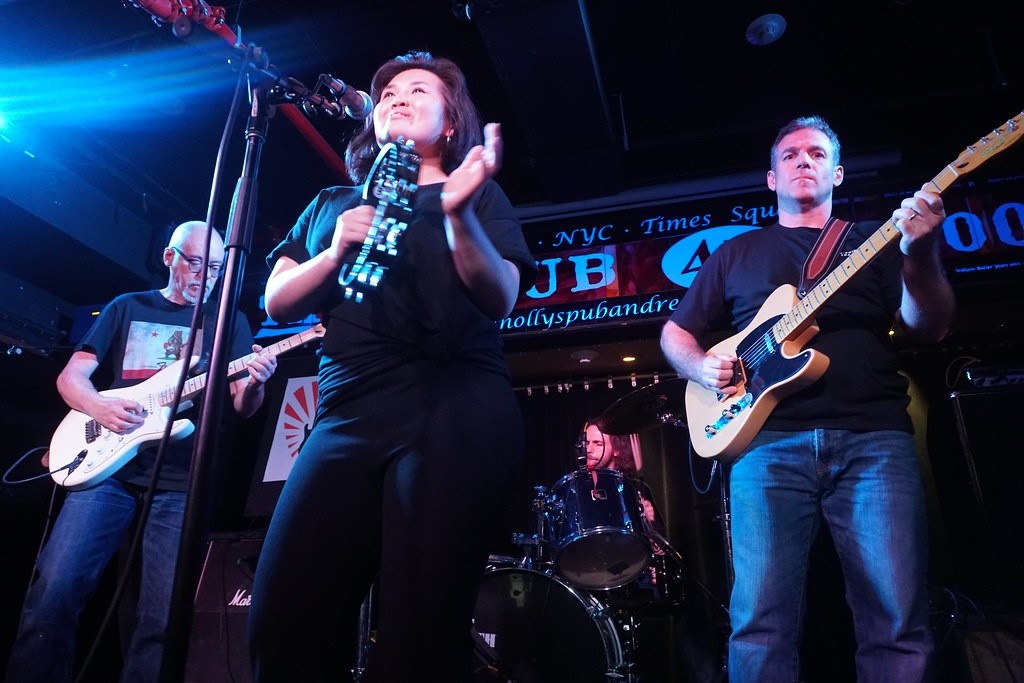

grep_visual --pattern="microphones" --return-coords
[318,73,373,120]
[580,422,588,465]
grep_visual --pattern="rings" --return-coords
[907,212,919,222]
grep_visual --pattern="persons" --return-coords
[660,116,955,683]
[8,219,278,682]
[244,52,536,683]
[581,418,657,527]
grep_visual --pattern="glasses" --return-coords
[170,247,224,278]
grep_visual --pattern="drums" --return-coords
[541,466,654,593]
[469,565,625,683]
[484,554,521,574]
[510,531,545,562]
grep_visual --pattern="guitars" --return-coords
[681,106,1024,469]
[46,323,326,494]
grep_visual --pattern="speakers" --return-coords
[927,384,1024,613]
[183,529,266,683]
